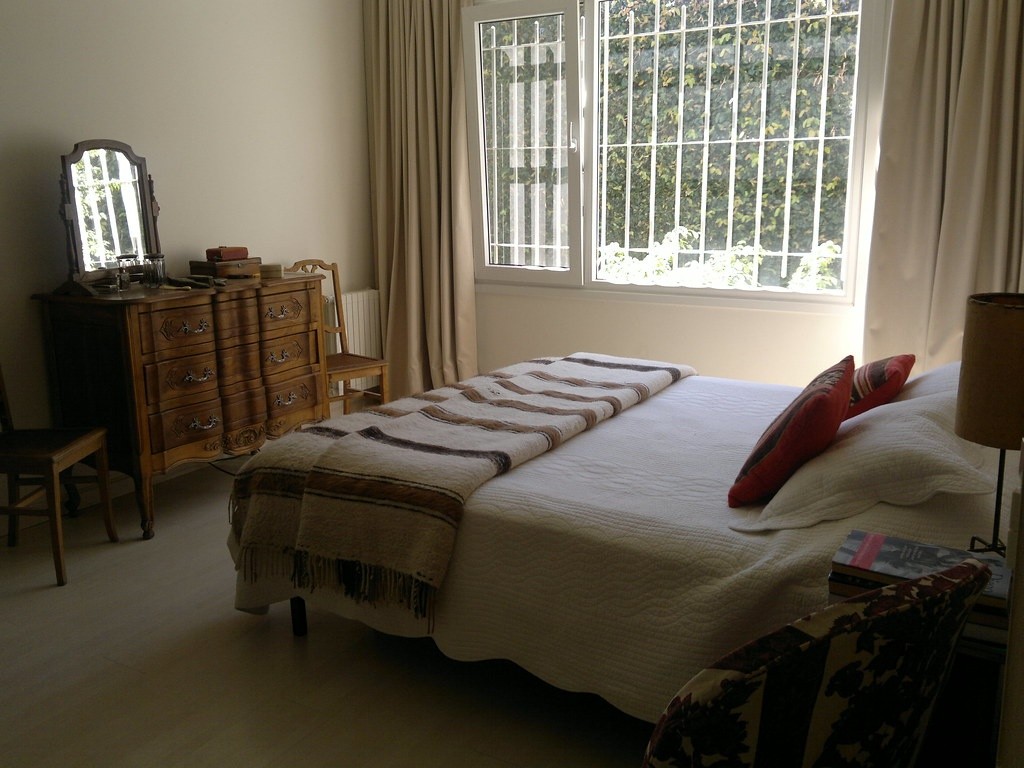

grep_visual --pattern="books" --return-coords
[826,527,1010,606]
[826,571,1009,630]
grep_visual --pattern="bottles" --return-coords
[116,268,131,293]
[143,253,165,289]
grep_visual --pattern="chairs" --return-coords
[643,557,995,767]
[1,377,121,584]
[283,259,389,416]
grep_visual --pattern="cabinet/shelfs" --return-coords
[30,269,331,539]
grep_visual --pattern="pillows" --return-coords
[729,355,855,509]
[894,359,963,403]
[842,354,917,421]
[727,387,996,532]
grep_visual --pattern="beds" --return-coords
[226,352,1024,727]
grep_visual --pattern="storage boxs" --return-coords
[190,246,262,279]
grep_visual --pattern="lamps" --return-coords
[956,291,1024,560]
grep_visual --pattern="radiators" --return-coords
[324,289,381,397]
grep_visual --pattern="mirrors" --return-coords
[61,139,164,284]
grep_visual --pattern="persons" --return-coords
[841,530,1005,585]
